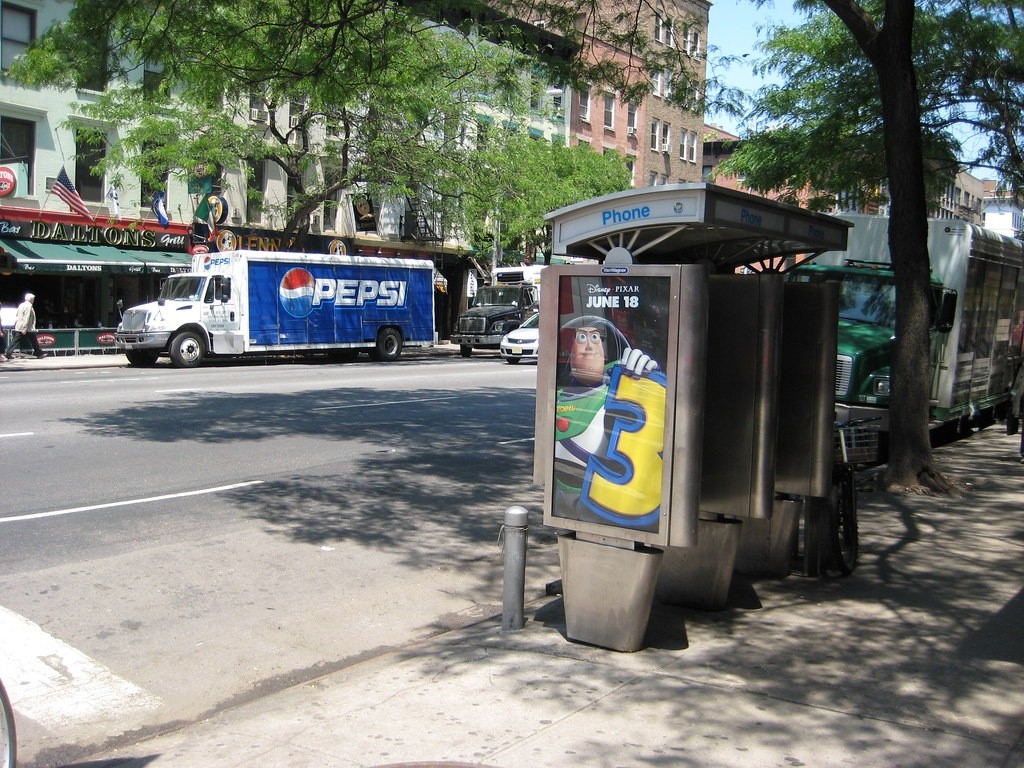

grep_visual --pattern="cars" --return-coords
[500,312,539,364]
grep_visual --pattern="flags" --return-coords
[51,166,95,224]
[193,191,219,243]
[152,188,169,229]
[106,185,123,221]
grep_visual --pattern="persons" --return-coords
[0,303,9,362]
[3,293,48,359]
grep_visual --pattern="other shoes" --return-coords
[37,354,47,359]
[5,354,14,360]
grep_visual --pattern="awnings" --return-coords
[0,236,197,277]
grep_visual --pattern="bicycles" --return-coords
[831,415,883,575]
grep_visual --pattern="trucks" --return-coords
[786,212,1023,450]
[114,249,438,369]
[450,264,547,358]
